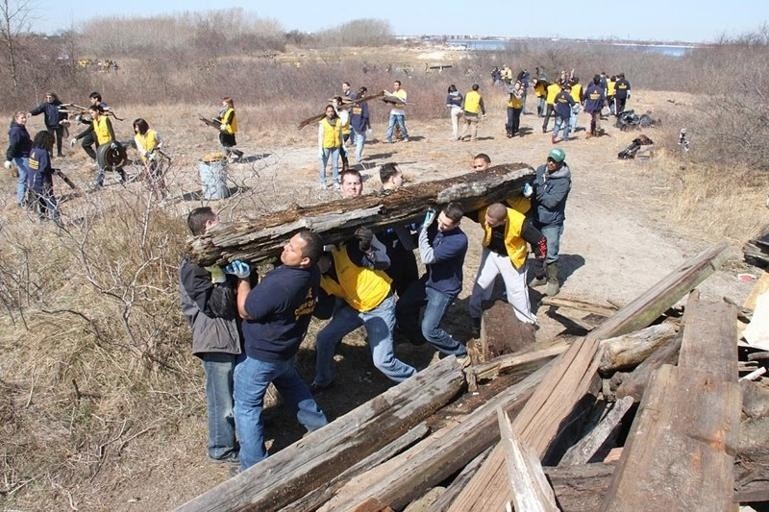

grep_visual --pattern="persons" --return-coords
[461,84,486,141]
[446,84,464,141]
[213,97,244,164]
[4,90,165,229]
[381,80,409,144]
[332,97,350,172]
[490,64,631,143]
[342,81,358,144]
[318,105,342,189]
[350,88,372,162]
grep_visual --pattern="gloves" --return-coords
[423,209,436,228]
[71,138,77,148]
[204,260,250,283]
[5,161,12,169]
[524,183,533,197]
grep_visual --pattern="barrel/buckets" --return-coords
[198,154,228,202]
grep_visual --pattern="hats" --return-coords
[548,148,565,163]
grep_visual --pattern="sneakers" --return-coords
[528,277,559,297]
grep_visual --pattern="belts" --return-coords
[366,280,396,312]
